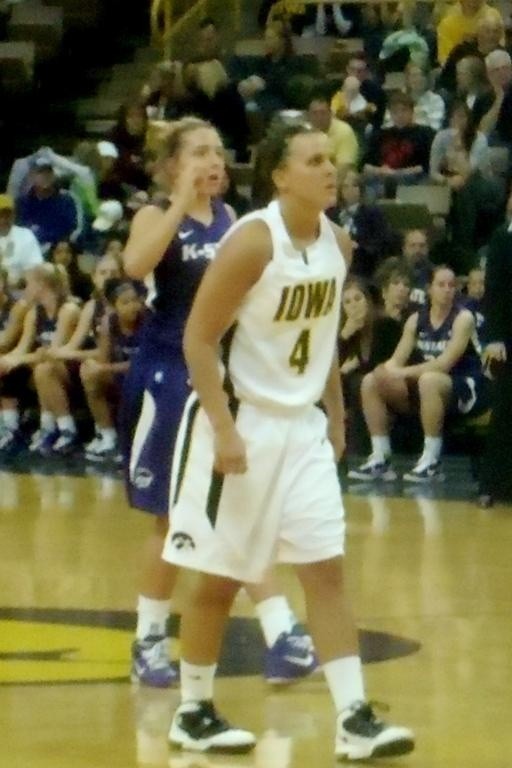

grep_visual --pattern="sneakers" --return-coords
[346,454,395,480]
[0,426,117,462]
[403,460,445,483]
[131,642,178,686]
[335,707,415,761]
[264,634,319,685]
[167,699,256,754]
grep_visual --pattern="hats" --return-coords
[92,200,126,233]
[30,147,60,169]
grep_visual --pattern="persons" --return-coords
[1,1,512,504]
[117,112,325,688]
[160,124,415,766]
